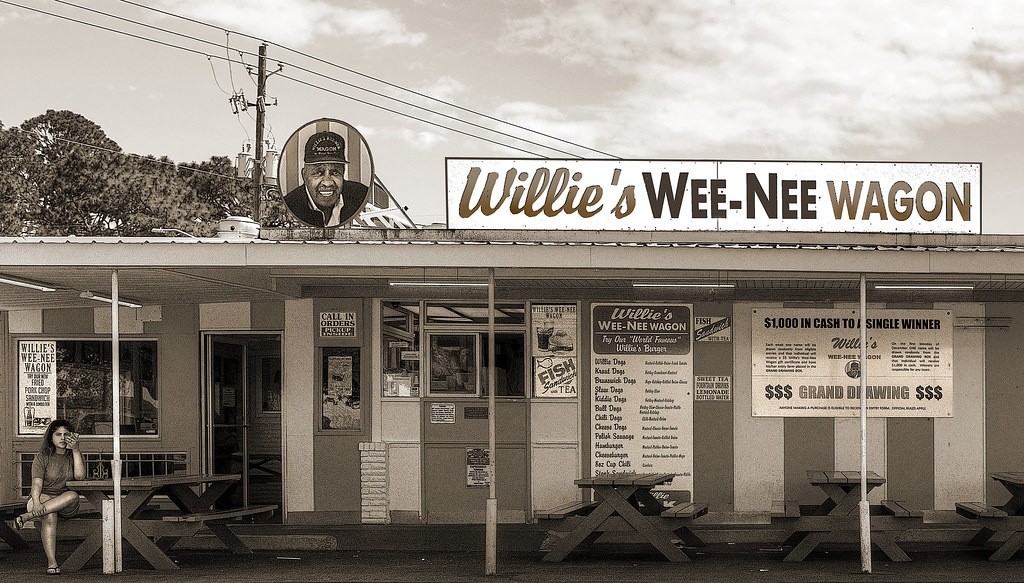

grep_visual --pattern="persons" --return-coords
[14,419,87,574]
[284,131,368,227]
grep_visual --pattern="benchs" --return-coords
[34,505,160,539]
[955,502,1009,525]
[770,500,801,531]
[533,501,600,531]
[162,503,284,538]
[661,502,709,530]
[881,501,924,530]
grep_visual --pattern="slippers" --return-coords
[46,567,62,575]
[14,515,25,531]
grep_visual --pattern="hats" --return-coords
[304,131,351,166]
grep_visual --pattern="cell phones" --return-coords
[66,432,79,449]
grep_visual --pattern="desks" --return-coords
[59,473,242,573]
[542,474,707,563]
[779,471,912,562]
[966,472,1024,562]
